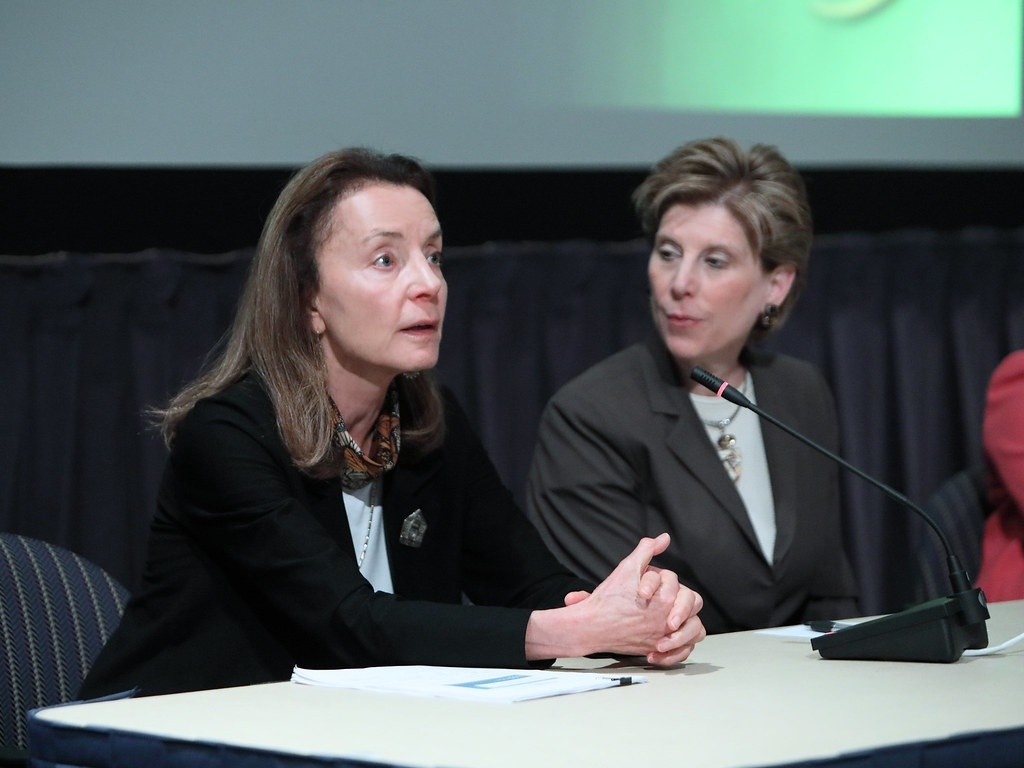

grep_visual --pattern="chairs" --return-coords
[0,531,134,768]
[918,463,1000,601]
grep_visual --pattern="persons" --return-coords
[523,139,859,634]
[972,349,1024,603]
[75,147,706,703]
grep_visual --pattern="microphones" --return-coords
[689,362,993,666]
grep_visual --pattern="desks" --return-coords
[28,599,1024,768]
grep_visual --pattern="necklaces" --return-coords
[703,372,747,450]
[358,480,376,568]
